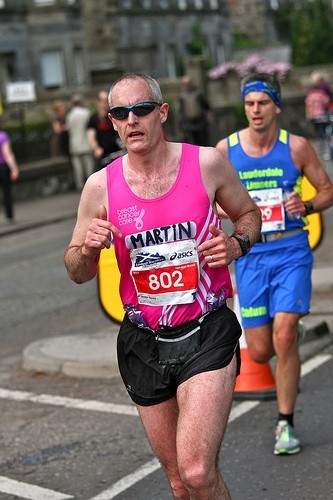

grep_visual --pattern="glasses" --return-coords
[108,100,162,120]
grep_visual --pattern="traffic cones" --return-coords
[228,288,279,400]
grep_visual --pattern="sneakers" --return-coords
[298,320,305,345]
[273,420,301,455]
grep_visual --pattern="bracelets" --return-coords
[303,201,314,217]
[230,232,251,257]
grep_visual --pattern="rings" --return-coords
[211,255,212,262]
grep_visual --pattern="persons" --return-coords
[176,75,215,146]
[0,113,19,226]
[64,92,104,190]
[303,70,332,159]
[212,73,333,455]
[84,89,124,172]
[64,72,262,500]
[49,98,67,157]
